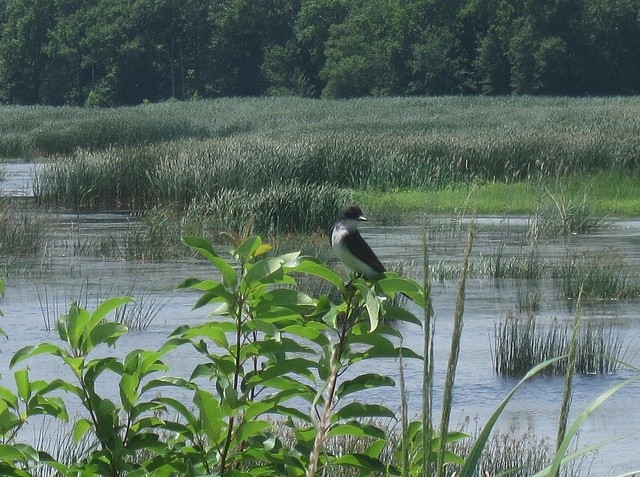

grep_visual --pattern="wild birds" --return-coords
[330,206,417,302]
